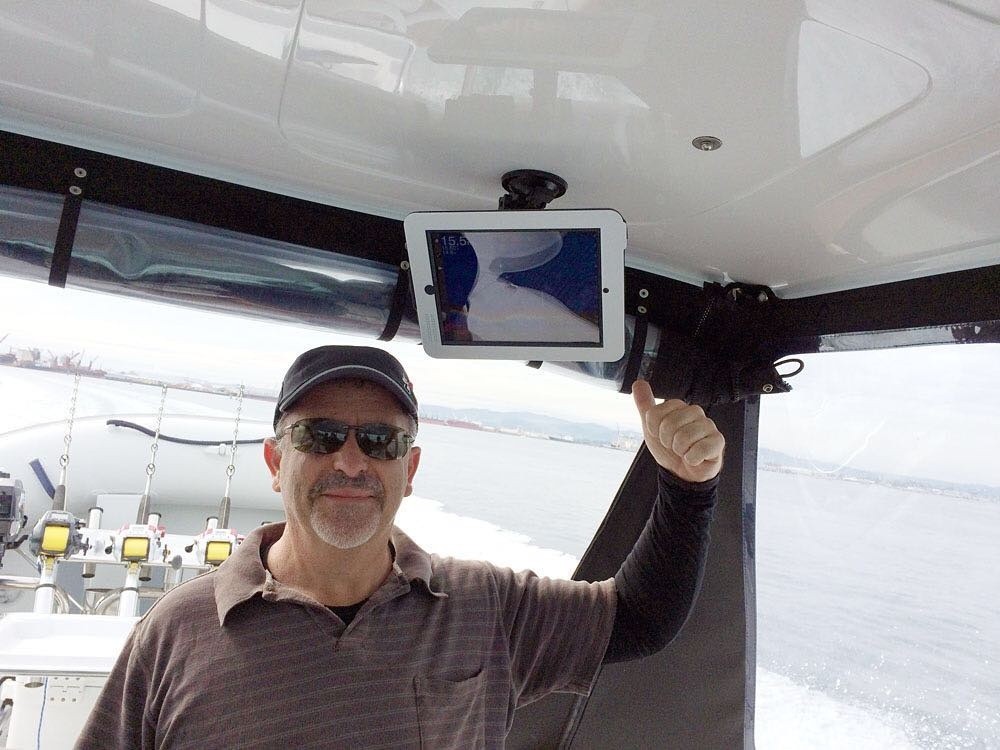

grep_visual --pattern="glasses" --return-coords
[282,416,415,461]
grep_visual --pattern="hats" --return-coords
[272,344,418,435]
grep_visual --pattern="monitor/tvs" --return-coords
[403,207,624,361]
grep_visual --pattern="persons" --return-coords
[74,345,725,750]
